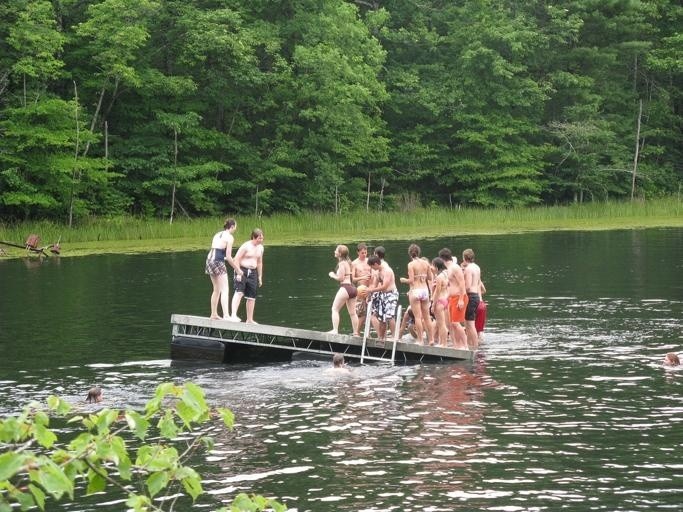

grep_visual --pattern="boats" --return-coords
[170,313,473,363]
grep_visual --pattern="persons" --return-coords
[204,216,245,324]
[327,353,349,371]
[324,240,486,353]
[662,352,681,365]
[85,385,103,404]
[228,228,264,327]
